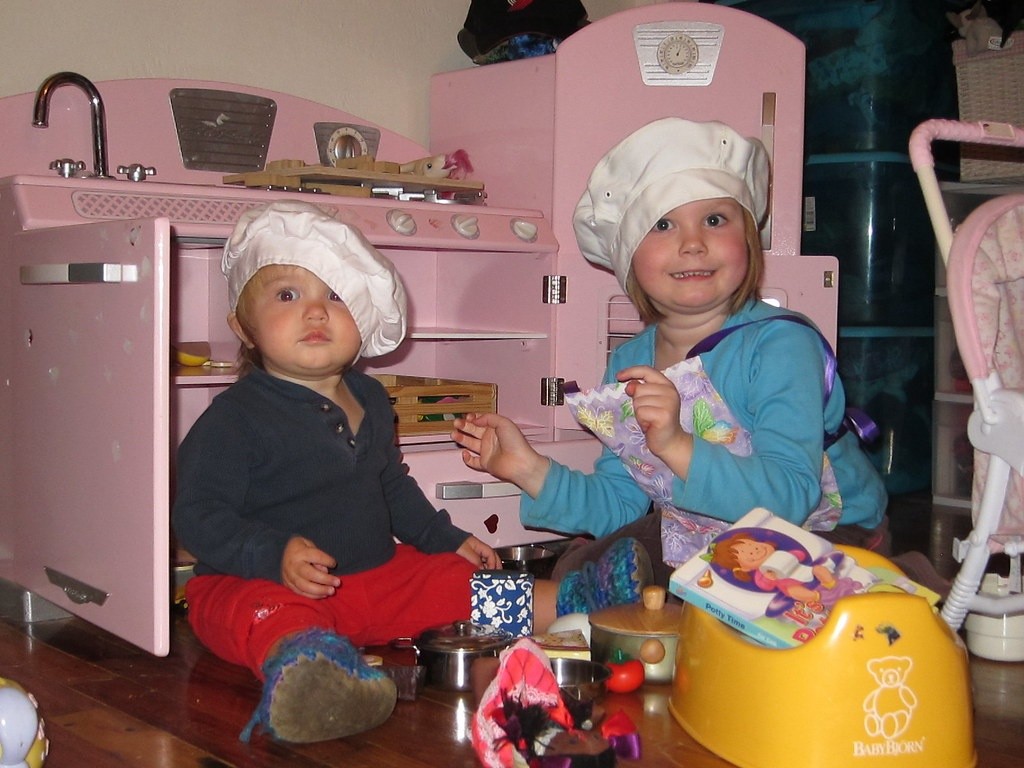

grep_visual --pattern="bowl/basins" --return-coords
[547,656,613,714]
[490,544,558,583]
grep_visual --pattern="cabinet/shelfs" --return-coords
[721,0,968,491]
[930,180,1024,581]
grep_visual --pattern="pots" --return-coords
[389,619,515,692]
[588,586,682,686]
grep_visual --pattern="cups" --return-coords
[469,568,535,640]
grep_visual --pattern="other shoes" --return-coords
[556,536,655,616]
[238,626,399,746]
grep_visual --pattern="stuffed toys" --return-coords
[469,636,641,768]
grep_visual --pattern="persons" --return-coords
[449,118,955,602]
[170,198,656,743]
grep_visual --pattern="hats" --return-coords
[220,199,407,368]
[572,116,770,298]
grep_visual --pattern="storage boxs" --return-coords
[368,373,499,437]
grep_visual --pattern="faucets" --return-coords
[30,71,157,180]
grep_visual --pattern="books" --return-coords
[668,506,943,651]
